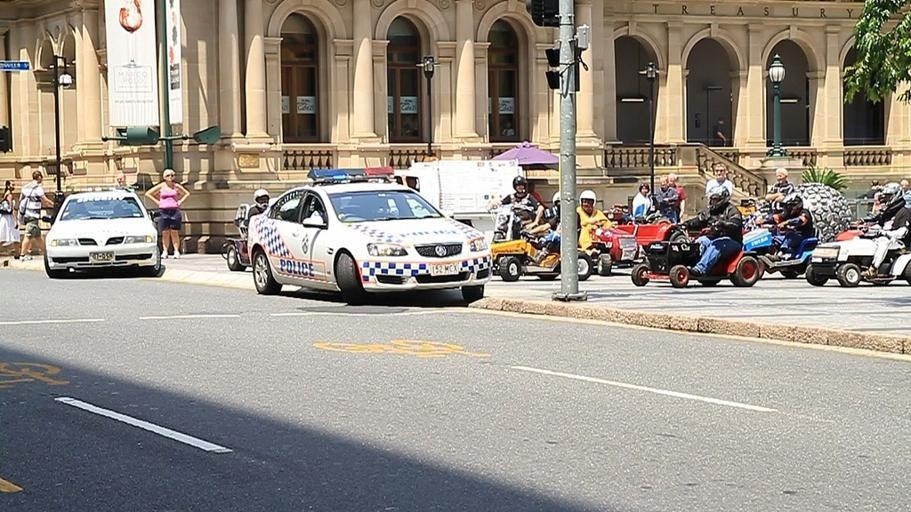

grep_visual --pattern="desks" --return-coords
[0,124,11,154]
[51,56,73,221]
[767,49,787,156]
[416,53,440,158]
[639,59,663,194]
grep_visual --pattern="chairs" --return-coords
[173,251,180,258]
[161,252,168,258]
[688,266,700,276]
[861,265,878,278]
[766,254,779,261]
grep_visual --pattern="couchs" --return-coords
[545,45,581,94]
[525,0,558,27]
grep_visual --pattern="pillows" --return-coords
[253,189,269,209]
[878,182,903,211]
[707,186,730,211]
[780,196,803,219]
[513,176,526,187]
[553,192,560,205]
[580,190,596,206]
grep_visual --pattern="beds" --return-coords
[491,141,581,179]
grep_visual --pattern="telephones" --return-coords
[0,60,31,71]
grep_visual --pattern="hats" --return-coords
[42,186,166,281]
[245,164,493,305]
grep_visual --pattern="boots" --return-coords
[220,199,259,275]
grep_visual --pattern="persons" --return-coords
[317,197,346,222]
[850,179,910,279]
[609,208,627,225]
[245,186,271,220]
[671,185,743,278]
[527,191,561,264]
[631,183,653,224]
[705,164,732,198]
[650,177,676,223]
[713,116,728,147]
[12,171,54,261]
[668,173,687,223]
[144,168,191,260]
[0,179,21,260]
[741,169,818,262]
[503,122,516,137]
[491,176,543,242]
[577,190,613,260]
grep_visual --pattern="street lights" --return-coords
[17,198,28,225]
[0,200,15,214]
